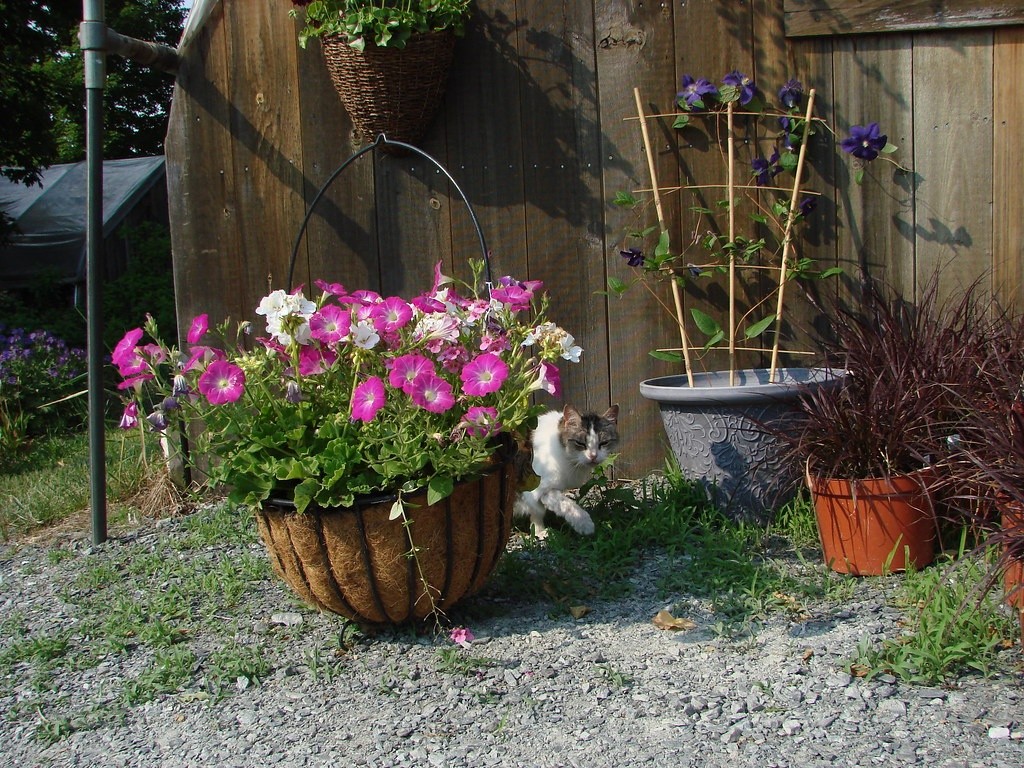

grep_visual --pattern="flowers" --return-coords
[110,254,585,648]
[591,68,919,367]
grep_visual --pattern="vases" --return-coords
[638,368,852,529]
[248,438,518,635]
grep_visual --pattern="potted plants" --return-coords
[703,343,964,578]
[896,389,1024,609]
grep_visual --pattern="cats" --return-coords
[513,403,621,538]
